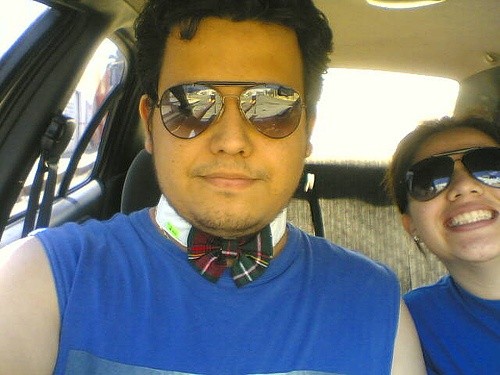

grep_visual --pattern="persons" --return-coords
[0,0,427,375]
[388,116,499,375]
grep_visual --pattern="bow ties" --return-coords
[183,226,275,287]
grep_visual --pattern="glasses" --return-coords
[407,146,500,202]
[155,80,309,139]
